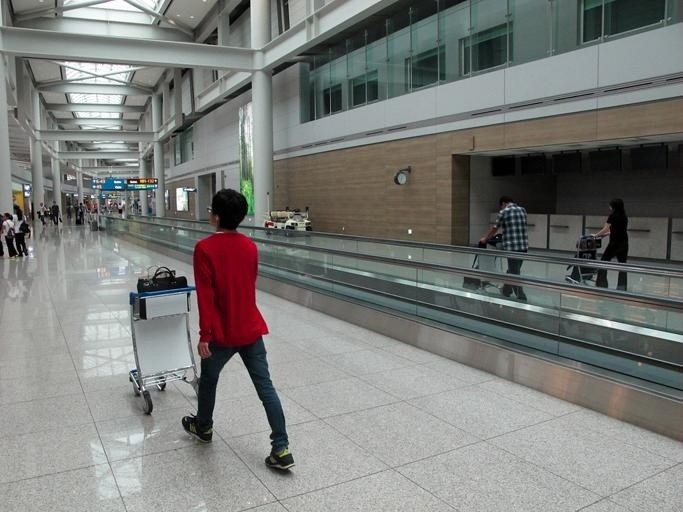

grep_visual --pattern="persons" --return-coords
[16,257,31,304]
[51,201,60,225]
[1,213,17,260]
[478,194,528,302]
[51,226,61,249]
[65,199,140,225]
[181,187,295,471]
[38,226,48,250]
[593,198,629,291]
[0,214,4,256]
[78,226,87,248]
[0,256,5,299]
[5,258,18,304]
[11,204,28,257]
[36,202,46,226]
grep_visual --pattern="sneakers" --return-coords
[9,249,29,258]
[264,446,297,471]
[181,413,213,444]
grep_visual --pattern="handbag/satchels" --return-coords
[575,236,602,249]
[19,220,30,234]
[478,233,505,249]
[136,264,188,321]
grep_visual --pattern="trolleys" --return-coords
[463,235,504,295]
[128,286,200,414]
[565,234,604,287]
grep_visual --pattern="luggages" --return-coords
[570,254,599,282]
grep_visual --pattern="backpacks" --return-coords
[5,220,16,240]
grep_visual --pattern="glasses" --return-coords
[204,202,216,214]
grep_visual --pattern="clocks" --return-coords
[393,170,407,185]
